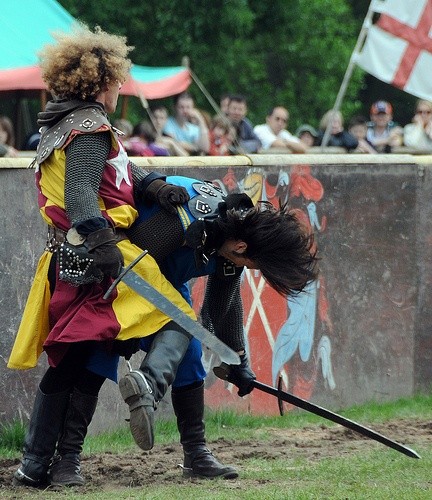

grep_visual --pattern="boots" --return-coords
[14,383,70,486]
[119,330,190,451]
[47,392,98,485]
[171,381,240,479]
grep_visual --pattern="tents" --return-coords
[0,0,246,156]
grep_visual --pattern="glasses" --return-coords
[415,109,432,115]
[272,114,288,126]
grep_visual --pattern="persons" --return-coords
[7,27,199,488]
[0,94,431,156]
[50,175,324,485]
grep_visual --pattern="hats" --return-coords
[370,101,392,115]
[295,123,319,139]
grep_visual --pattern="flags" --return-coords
[356,0,432,102]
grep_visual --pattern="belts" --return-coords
[46,226,69,245]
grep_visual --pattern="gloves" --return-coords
[116,339,140,361]
[146,177,190,214]
[222,351,256,397]
[76,217,124,281]
